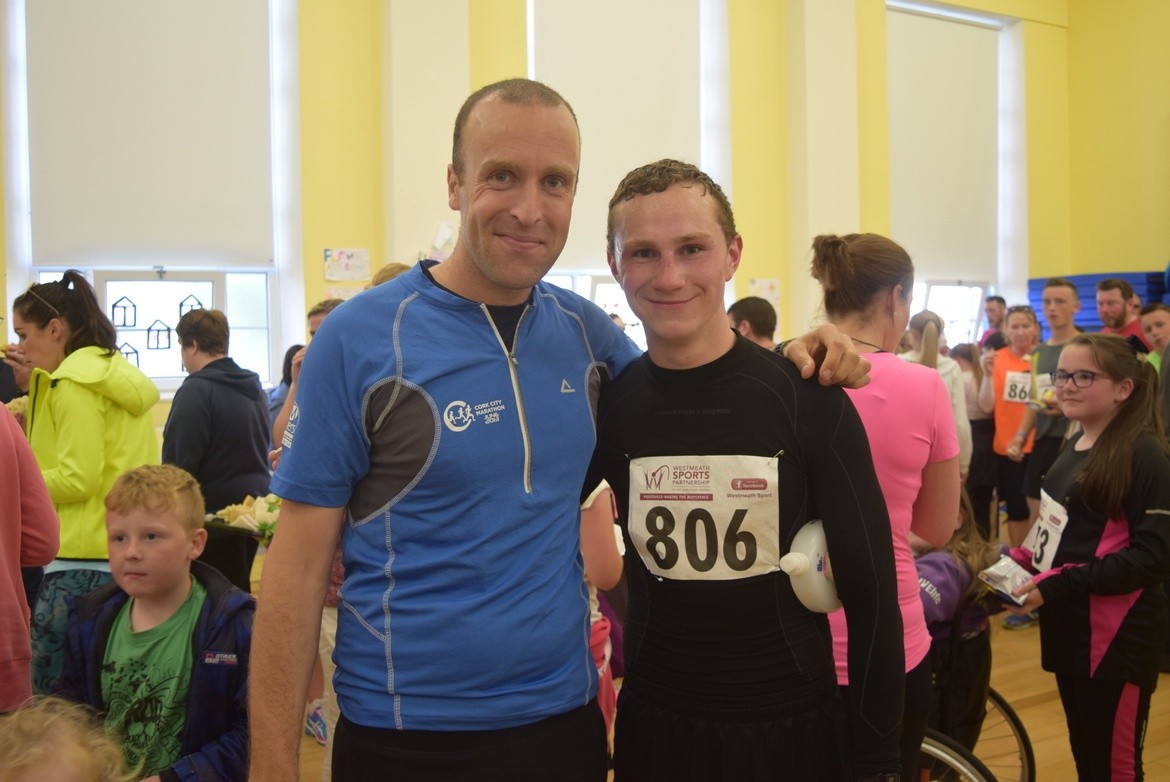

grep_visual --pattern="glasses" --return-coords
[1008,306,1033,313]
[1049,368,1121,389]
[24,282,62,322]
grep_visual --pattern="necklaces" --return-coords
[852,338,882,352]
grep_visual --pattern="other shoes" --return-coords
[997,500,1008,523]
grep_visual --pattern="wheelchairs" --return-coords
[914,646,1036,782]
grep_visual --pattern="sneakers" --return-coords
[1002,612,1039,631]
[304,707,327,746]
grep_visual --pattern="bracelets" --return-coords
[774,338,793,356]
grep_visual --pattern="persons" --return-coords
[0,77,1169,782]
